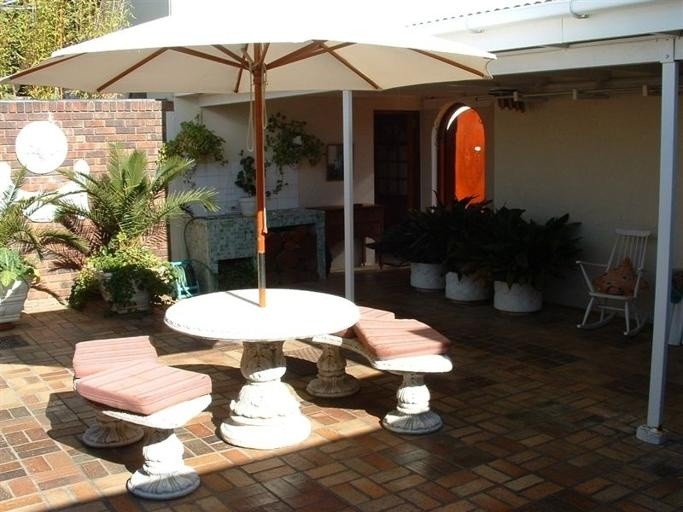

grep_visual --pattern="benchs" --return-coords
[309,300,456,436]
[71,334,213,500]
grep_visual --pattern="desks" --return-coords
[161,288,362,451]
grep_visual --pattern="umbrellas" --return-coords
[0,0,497,305]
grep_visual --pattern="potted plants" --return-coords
[0,165,47,325]
[234,156,271,215]
[388,194,576,314]
[49,144,221,319]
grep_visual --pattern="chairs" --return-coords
[576,224,653,340]
